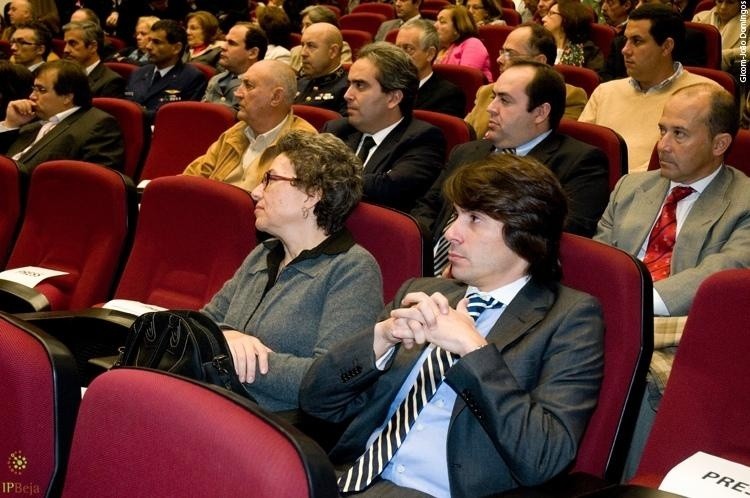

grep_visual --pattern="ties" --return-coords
[432,147,517,279]
[642,185,695,284]
[334,291,504,494]
[149,71,161,90]
[357,136,376,165]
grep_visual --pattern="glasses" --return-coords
[32,86,52,95]
[498,50,535,60]
[546,10,563,19]
[9,37,36,47]
[261,172,298,190]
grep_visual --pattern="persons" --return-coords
[199,130,385,425]
[2,1,749,205]
[588,81,750,484]
[299,153,607,494]
[407,58,611,277]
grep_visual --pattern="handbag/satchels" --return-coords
[114,307,244,396]
